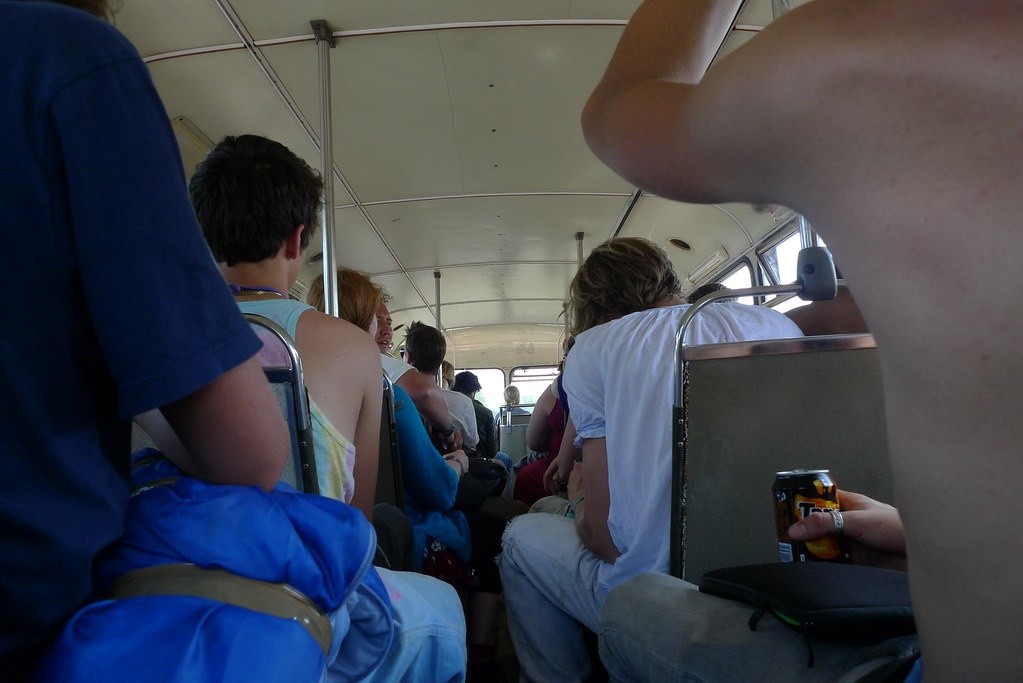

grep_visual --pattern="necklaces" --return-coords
[229,284,286,297]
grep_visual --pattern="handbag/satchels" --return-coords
[457,457,507,519]
[699,558,923,669]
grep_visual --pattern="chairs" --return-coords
[128,277,895,683]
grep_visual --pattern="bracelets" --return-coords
[442,424,455,437]
[571,493,584,512]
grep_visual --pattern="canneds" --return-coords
[772,468,843,565]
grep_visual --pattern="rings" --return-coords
[830,511,844,535]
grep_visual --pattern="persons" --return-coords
[581,2,1023,683]
[136,135,466,682]
[0,0,289,683]
[306,237,870,683]
